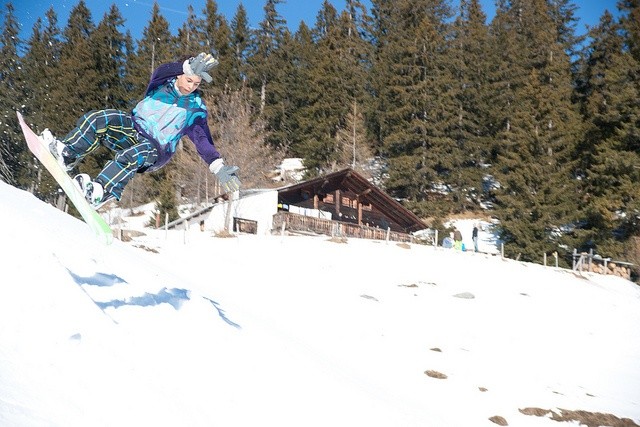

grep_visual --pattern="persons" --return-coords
[41,52,243,211]
[454,230,463,252]
[472,227,479,252]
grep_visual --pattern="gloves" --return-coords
[209,158,242,194]
[183,52,219,83]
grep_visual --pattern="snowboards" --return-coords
[14,111,112,235]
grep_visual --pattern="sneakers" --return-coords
[75,172,114,204]
[42,128,75,167]
